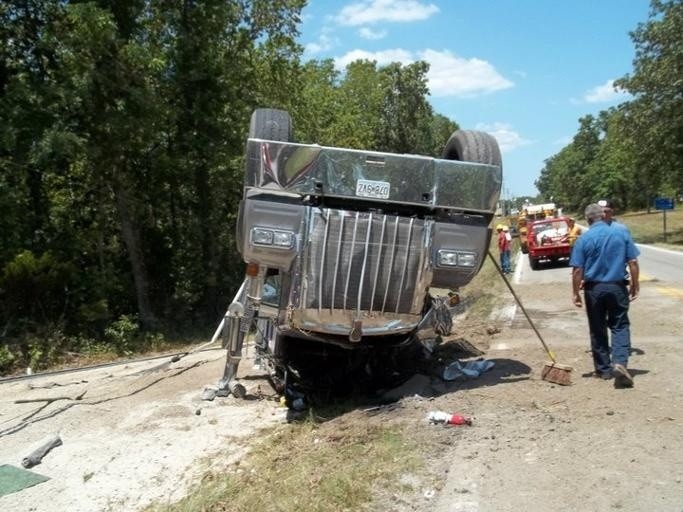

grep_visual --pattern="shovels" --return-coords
[16,390,88,404]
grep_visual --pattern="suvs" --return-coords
[211,106,503,413]
[515,202,582,270]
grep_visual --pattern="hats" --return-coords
[597,199,614,210]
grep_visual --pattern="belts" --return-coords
[586,278,631,286]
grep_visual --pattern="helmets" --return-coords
[496,224,503,229]
[503,225,509,232]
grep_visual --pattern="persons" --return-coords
[495,225,509,274]
[560,216,583,257]
[502,227,511,252]
[578,199,630,290]
[567,202,641,390]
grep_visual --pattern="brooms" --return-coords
[486,247,575,388]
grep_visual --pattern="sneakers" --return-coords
[613,361,635,387]
[591,369,612,379]
